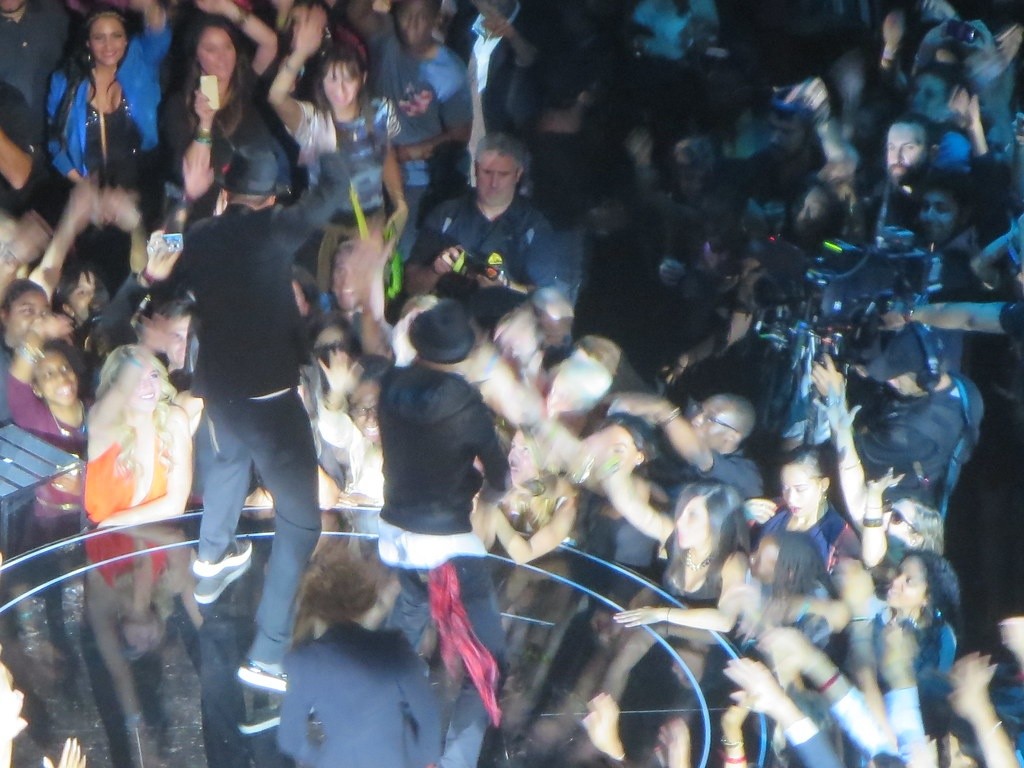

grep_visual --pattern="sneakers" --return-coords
[237,657,287,693]
[194,556,252,605]
[192,536,253,576]
[237,698,281,734]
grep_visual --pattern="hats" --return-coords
[409,303,476,365]
[866,328,928,382]
[215,143,281,194]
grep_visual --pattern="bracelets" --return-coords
[654,407,681,428]
[822,396,846,404]
[819,672,839,692]
[617,752,625,762]
[236,9,248,27]
[863,518,884,527]
[722,754,746,763]
[17,343,40,365]
[666,607,671,622]
[869,507,883,509]
[843,459,861,470]
[284,56,298,71]
[192,126,212,144]
[720,738,743,747]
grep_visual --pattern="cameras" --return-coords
[163,233,183,252]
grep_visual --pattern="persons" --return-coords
[0,1,1024,768]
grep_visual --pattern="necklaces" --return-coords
[50,400,87,438]
[684,551,714,571]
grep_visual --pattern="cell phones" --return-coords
[200,75,220,111]
[946,20,975,43]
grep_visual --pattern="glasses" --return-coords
[355,403,380,417]
[889,508,917,534]
[690,402,741,437]
[91,30,128,41]
